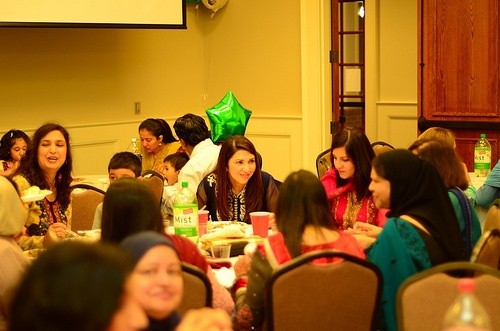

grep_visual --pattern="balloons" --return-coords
[205,91,252,143]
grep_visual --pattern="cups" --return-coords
[211,244,230,261]
[164,220,170,232]
[249,211,270,238]
[199,209,209,237]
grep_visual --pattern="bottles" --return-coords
[175,181,198,246]
[474,134,492,178]
[127,138,143,157]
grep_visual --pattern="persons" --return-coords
[235,171,367,331]
[408,127,481,262]
[319,126,389,230]
[474,159,500,209]
[197,137,279,224]
[0,115,235,331]
[367,149,475,331]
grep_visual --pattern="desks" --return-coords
[69,171,167,192]
[24,220,375,291]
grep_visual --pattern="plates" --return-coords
[19,189,53,202]
[24,249,47,261]
[86,230,103,237]
[211,221,248,234]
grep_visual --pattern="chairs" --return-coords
[69,140,500,331]
